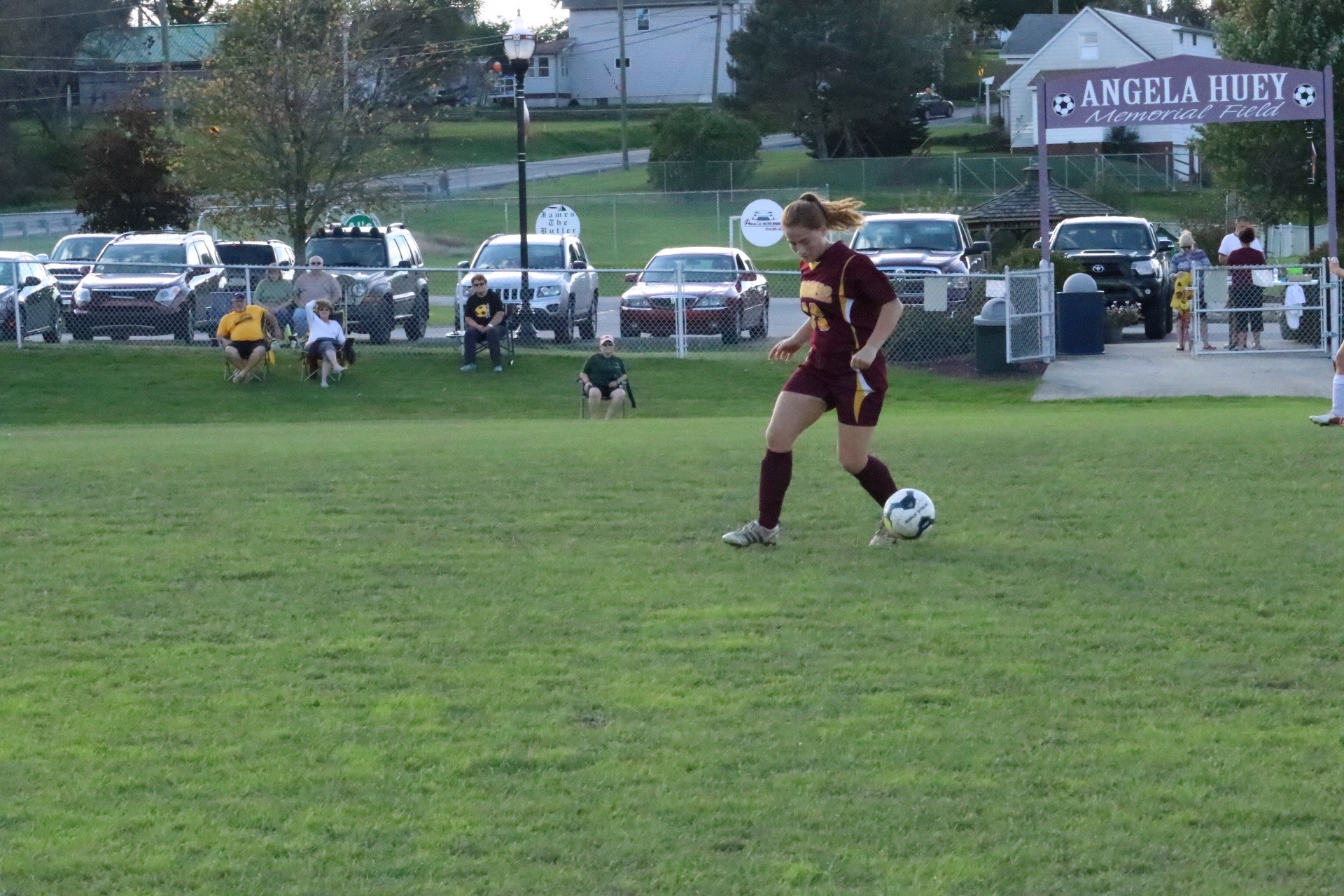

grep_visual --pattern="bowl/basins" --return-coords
[1287,274,1312,282]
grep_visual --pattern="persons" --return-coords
[579,334,629,419]
[253,263,293,332]
[1167,228,1214,352]
[460,274,507,372]
[293,255,342,337]
[306,299,346,389]
[720,191,904,547]
[216,291,282,386]
[1218,215,1267,352]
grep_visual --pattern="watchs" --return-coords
[1309,257,1344,425]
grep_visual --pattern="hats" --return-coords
[600,335,614,346]
[232,292,245,299]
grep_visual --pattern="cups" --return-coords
[1280,278,1288,282]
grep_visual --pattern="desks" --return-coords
[1227,280,1318,286]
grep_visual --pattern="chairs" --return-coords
[576,378,628,418]
[1073,230,1095,249]
[1121,229,1141,250]
[464,305,514,367]
[300,310,346,384]
[223,313,270,382]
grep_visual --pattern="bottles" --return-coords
[1273,267,1279,285]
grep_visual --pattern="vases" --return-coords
[1106,324,1124,343]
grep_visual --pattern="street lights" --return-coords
[501,6,545,348]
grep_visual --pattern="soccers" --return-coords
[1052,92,1075,117]
[1294,84,1316,107]
[884,488,936,540]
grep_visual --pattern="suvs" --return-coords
[303,210,431,345]
[212,238,295,291]
[35,232,123,333]
[453,232,599,344]
[847,212,992,361]
[68,229,231,344]
[1032,212,1176,340]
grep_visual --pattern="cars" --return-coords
[618,247,771,346]
[0,250,65,344]
[910,90,954,121]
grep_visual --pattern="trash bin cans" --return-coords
[973,298,1021,373]
[1056,272,1106,355]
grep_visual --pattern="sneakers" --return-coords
[1309,413,1344,427]
[722,521,780,548]
[868,515,898,548]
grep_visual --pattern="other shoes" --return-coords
[1203,346,1216,351]
[495,366,502,372]
[1176,346,1184,351]
[233,369,244,385]
[240,373,253,384]
[460,364,473,371]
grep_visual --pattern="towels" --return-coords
[1284,286,1305,331]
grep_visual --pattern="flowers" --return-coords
[1105,301,1145,324]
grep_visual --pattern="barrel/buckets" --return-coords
[1287,267,1304,277]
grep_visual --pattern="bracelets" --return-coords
[616,380,622,386]
[487,324,493,328]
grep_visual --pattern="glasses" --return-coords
[474,283,484,287]
[311,261,322,266]
[320,308,329,311]
[603,344,613,347]
[1181,246,1192,249]
